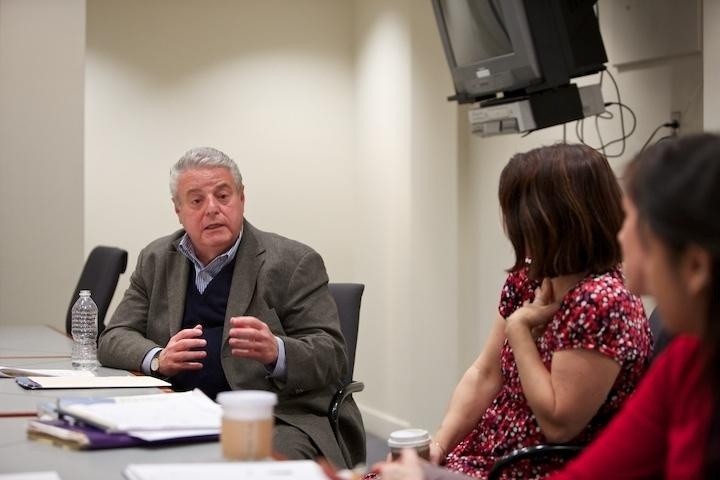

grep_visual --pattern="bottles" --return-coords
[71,288,100,371]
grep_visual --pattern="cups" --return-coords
[215,388,278,462]
[385,428,429,464]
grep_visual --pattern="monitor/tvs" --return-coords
[428,0,609,110]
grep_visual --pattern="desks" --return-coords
[0,321,74,358]
[0,417,280,480]
[0,358,180,417]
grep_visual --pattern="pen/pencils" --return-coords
[1,412,37,418]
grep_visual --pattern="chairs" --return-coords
[320,283,363,470]
[65,245,128,343]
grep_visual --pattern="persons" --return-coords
[345,132,720,480]
[98,147,366,480]
[428,142,655,480]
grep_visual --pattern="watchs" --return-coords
[150,350,161,378]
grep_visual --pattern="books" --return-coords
[25,419,218,449]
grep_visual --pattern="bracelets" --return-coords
[433,440,446,456]
